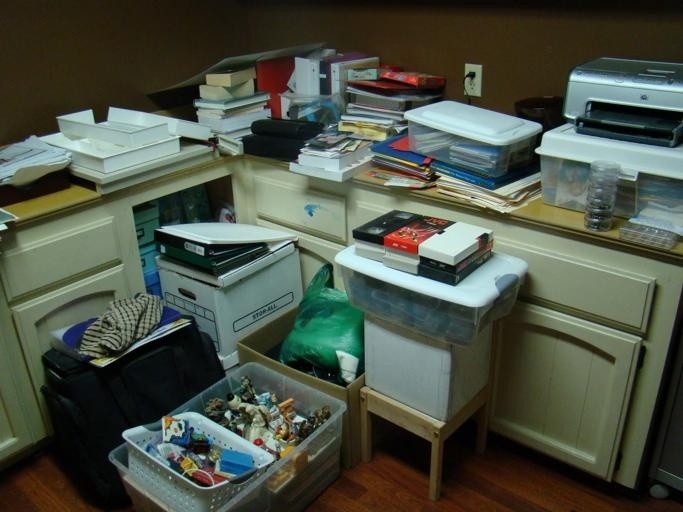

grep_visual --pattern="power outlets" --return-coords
[462,63,482,97]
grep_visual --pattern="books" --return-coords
[0,134,68,186]
[189,66,272,143]
[289,71,446,183]
[370,131,543,214]
[291,56,381,96]
[350,208,495,287]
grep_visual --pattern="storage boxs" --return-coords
[106,363,348,511]
[403,100,543,179]
[534,123,681,236]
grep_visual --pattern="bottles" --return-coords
[584,159,622,233]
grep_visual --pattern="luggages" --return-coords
[41,312,226,511]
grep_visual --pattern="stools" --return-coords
[359,383,494,502]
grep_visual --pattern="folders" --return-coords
[154,229,269,277]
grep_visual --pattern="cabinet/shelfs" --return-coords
[1,156,682,490]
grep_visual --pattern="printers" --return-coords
[562,55,683,149]
